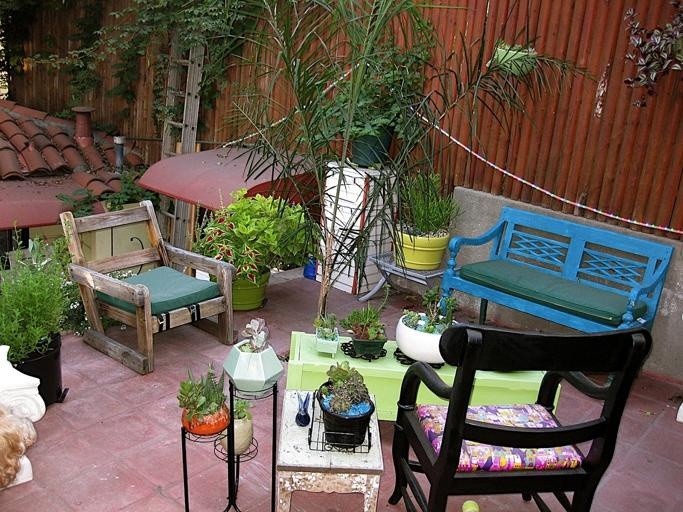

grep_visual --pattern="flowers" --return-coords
[191,189,321,287]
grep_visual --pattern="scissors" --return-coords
[295,392,311,428]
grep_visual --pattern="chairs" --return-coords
[59,200,236,374]
[387,325,653,512]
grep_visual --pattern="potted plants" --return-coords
[394,174,465,271]
[313,313,340,359]
[221,398,254,455]
[295,18,438,166]
[176,362,230,436]
[395,286,459,364]
[222,317,284,396]
[494,38,536,77]
[316,360,375,449]
[339,302,388,354]
[0,220,81,406]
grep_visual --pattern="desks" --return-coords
[286,331,561,421]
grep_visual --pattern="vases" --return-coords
[232,267,271,310]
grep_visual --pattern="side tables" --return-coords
[276,389,385,512]
[358,252,461,302]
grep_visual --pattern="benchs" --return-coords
[440,207,674,385]
